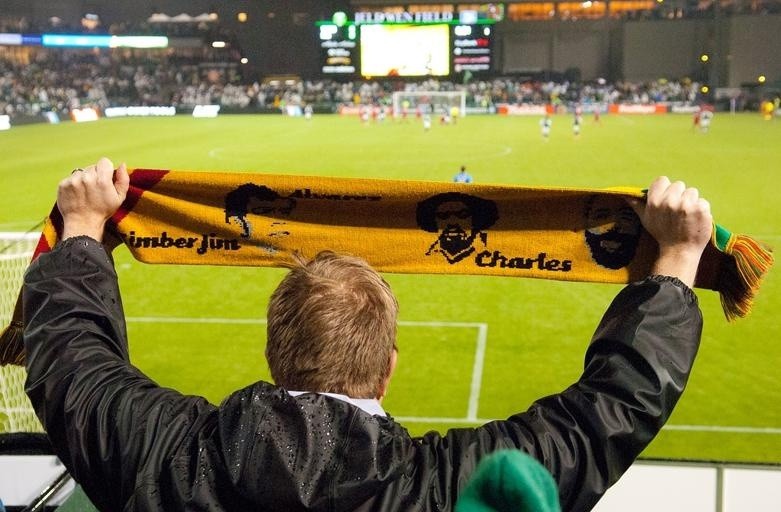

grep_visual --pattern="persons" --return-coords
[18,159,713,510]
[454,165,473,184]
[248,75,714,136]
[0,14,260,117]
[453,447,560,512]
[759,93,781,121]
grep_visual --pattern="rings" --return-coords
[72,167,85,174]
